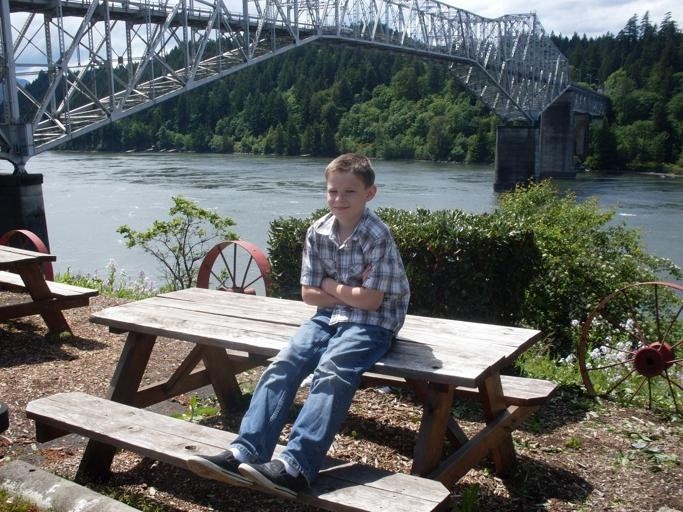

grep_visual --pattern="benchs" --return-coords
[0,269,98,311]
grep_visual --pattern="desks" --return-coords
[77,285,543,512]
[0,245,88,343]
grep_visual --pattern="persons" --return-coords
[184,151,412,501]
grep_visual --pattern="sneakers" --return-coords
[238,458,301,500]
[186,450,256,489]
